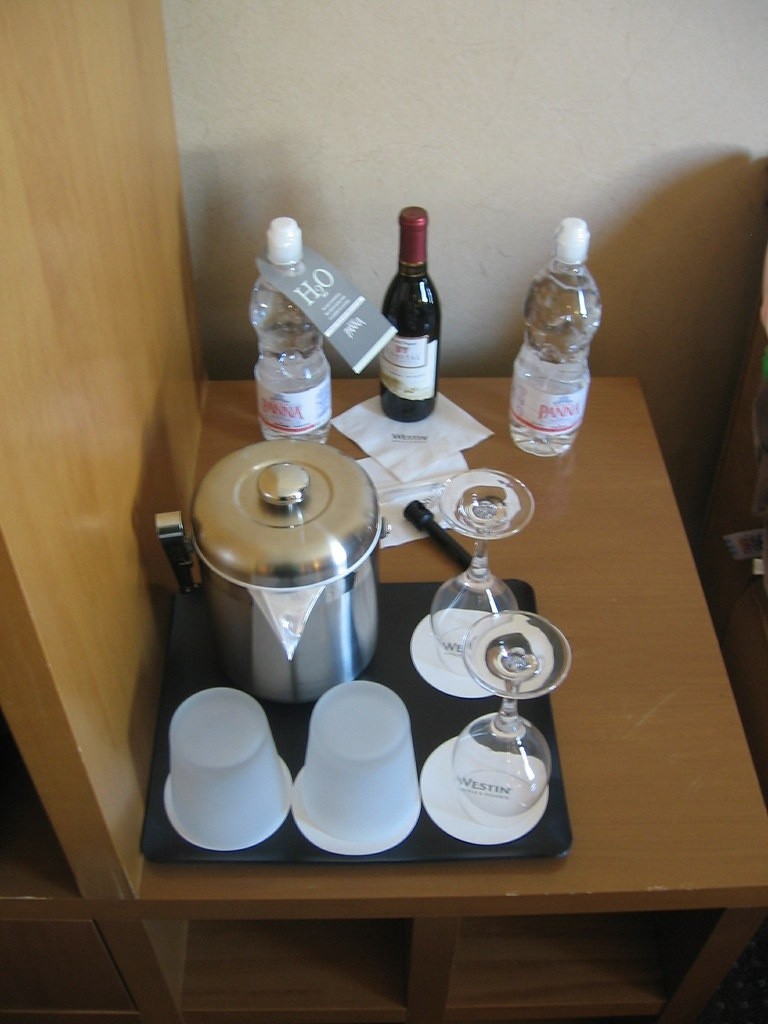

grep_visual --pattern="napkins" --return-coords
[354,452,478,547]
[329,390,494,484]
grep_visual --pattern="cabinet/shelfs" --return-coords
[0,1,768,1024]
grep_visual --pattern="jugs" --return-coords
[155,440,383,704]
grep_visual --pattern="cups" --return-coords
[162,686,292,854]
[289,678,421,855]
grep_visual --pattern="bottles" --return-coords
[508,218,604,456]
[376,206,443,423]
[247,217,333,445]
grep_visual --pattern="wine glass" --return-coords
[448,609,572,827]
[432,469,536,677]
